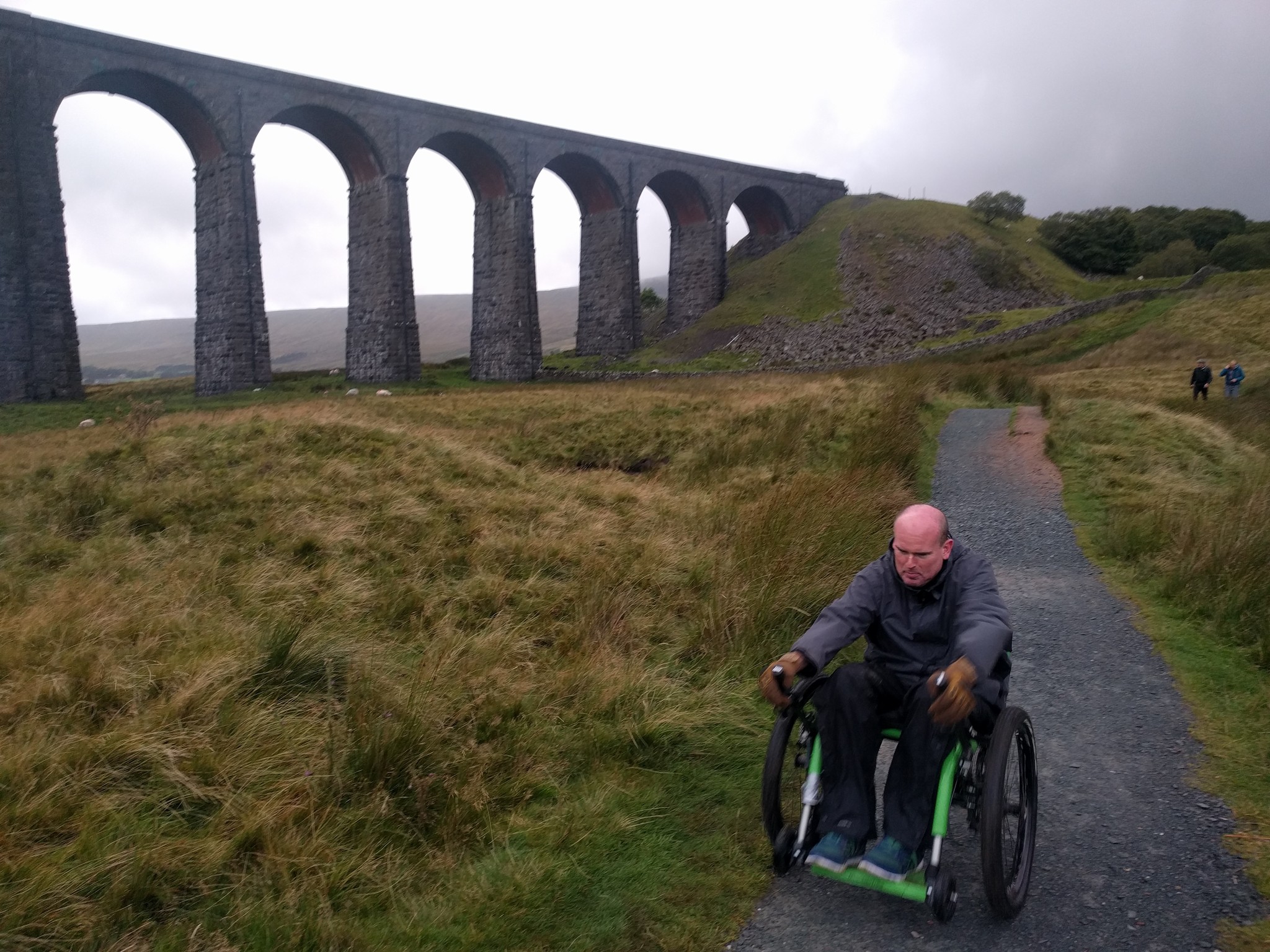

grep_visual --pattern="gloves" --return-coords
[925,656,977,736]
[759,652,807,705]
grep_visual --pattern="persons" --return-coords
[756,503,1013,881]
[1188,359,1213,400]
[1219,359,1245,397]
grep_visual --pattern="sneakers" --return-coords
[804,832,865,874]
[857,836,926,882]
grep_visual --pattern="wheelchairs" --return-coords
[759,672,1041,925]
